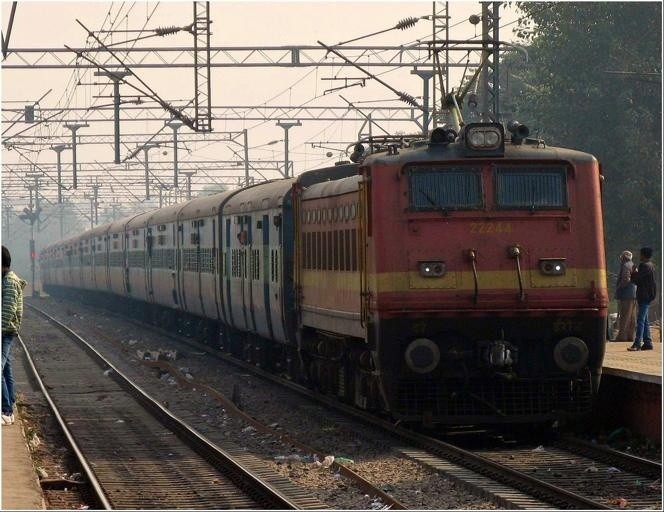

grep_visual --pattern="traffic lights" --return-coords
[28,239,35,261]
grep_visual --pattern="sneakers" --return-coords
[1,414,13,425]
[627,344,641,351]
[11,401,17,412]
[641,342,652,349]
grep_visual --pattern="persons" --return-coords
[609,250,638,342]
[626,247,656,350]
[2,246,29,425]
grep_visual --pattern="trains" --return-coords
[38,117,609,445]
